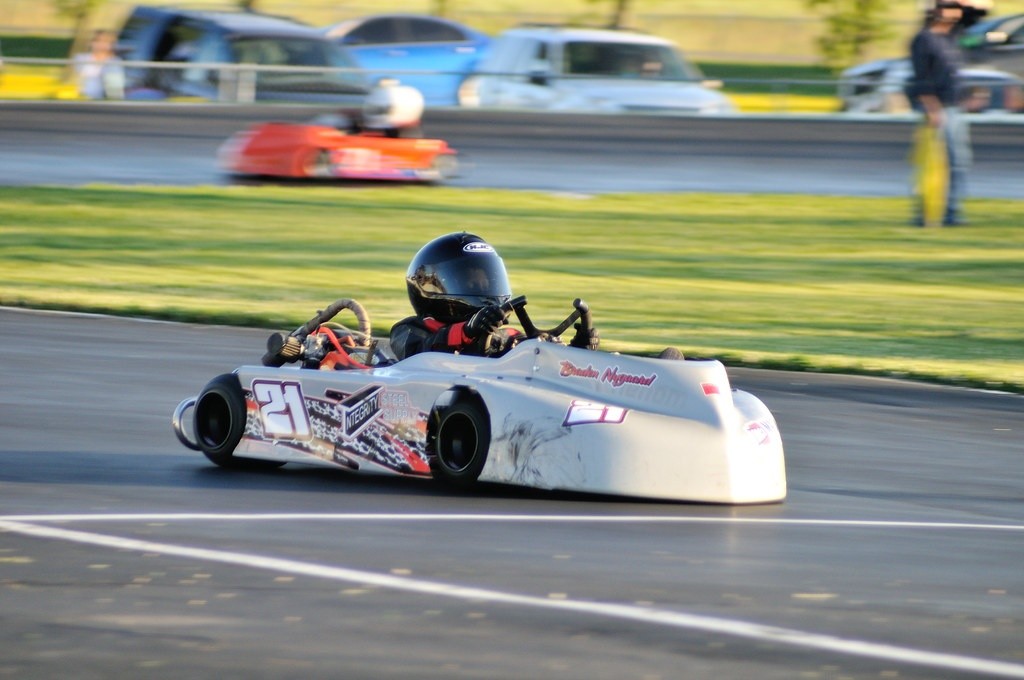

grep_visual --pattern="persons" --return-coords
[71,27,125,101]
[351,79,424,139]
[388,231,686,360]
[902,1,990,226]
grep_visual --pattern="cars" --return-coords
[848,64,1024,115]
[322,13,501,107]
[839,10,1024,108]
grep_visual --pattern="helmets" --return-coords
[407,231,511,323]
[362,79,424,129]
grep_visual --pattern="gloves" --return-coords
[570,322,599,350]
[464,304,508,337]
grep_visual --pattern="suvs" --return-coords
[462,29,737,117]
[114,2,377,103]
